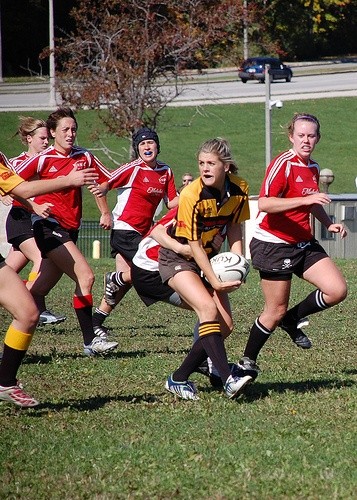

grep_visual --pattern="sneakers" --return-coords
[93,325,113,339]
[236,357,262,372]
[103,272,120,308]
[163,374,200,401]
[232,365,258,382]
[194,360,209,376]
[278,317,312,349]
[83,337,119,356]
[0,379,39,407]
[36,309,66,326]
[223,375,253,398]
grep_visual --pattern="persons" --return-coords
[0,106,259,408]
[240,113,349,370]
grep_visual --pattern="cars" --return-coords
[238,56,293,84]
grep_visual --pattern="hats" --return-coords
[132,128,159,155]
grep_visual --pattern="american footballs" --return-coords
[200,252,252,286]
